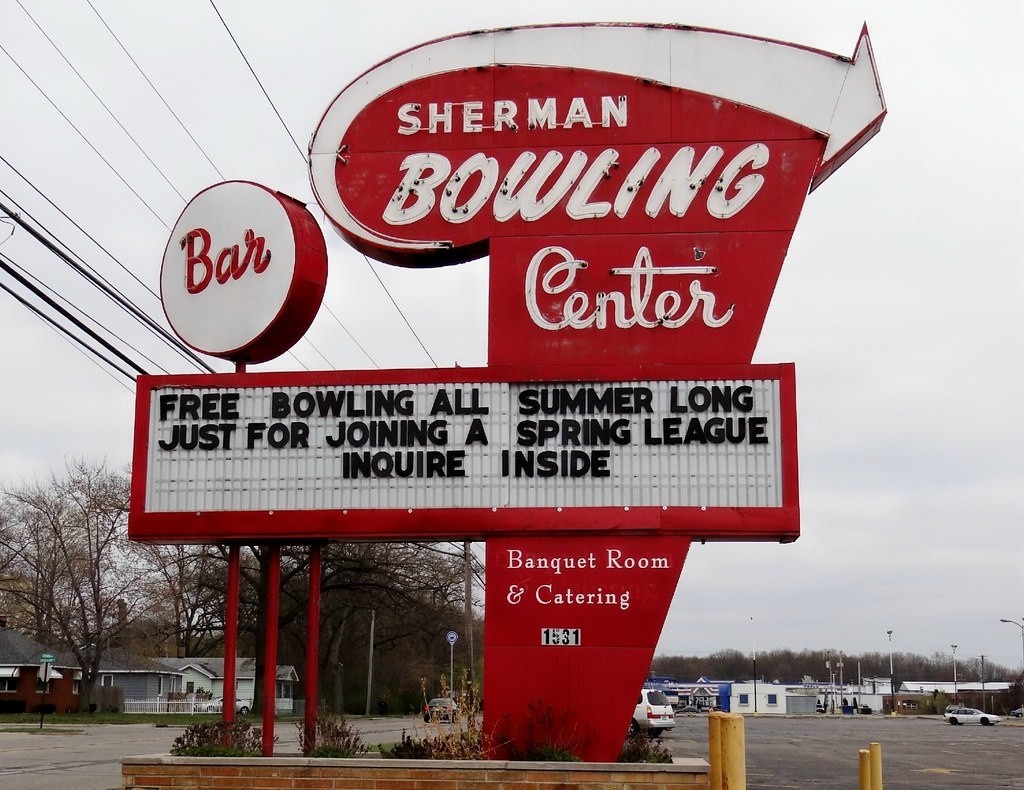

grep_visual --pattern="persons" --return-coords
[697,701,701,713]
[853,697,857,706]
[843,698,848,705]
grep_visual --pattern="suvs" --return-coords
[626,689,676,737]
[944,705,968,717]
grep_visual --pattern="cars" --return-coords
[194,694,251,716]
[1010,708,1024,718]
[424,698,458,723]
[816,704,825,713]
[675,705,702,714]
[943,707,1002,726]
[701,705,722,712]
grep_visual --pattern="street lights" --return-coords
[951,645,957,705]
[887,630,897,716]
[1000,619,1024,671]
